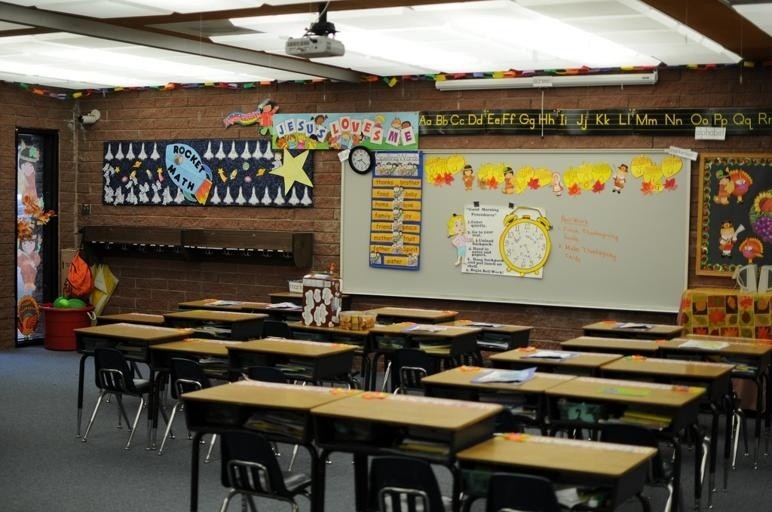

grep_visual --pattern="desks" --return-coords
[680,288,772,340]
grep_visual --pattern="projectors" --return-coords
[284,35,346,60]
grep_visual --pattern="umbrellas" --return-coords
[88,260,119,326]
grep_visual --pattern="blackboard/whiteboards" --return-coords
[339,148,692,313]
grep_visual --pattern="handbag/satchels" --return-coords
[64,250,93,297]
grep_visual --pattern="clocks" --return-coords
[498,203,552,275]
[348,145,371,175]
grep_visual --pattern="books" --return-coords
[477,332,510,351]
[379,335,453,355]
[376,424,452,460]
[247,410,304,442]
[195,354,229,380]
[270,357,312,380]
[195,322,230,336]
[295,329,364,355]
[117,340,144,361]
[620,404,669,430]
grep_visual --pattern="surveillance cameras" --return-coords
[76,113,97,126]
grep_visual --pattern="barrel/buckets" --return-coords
[39,304,95,351]
